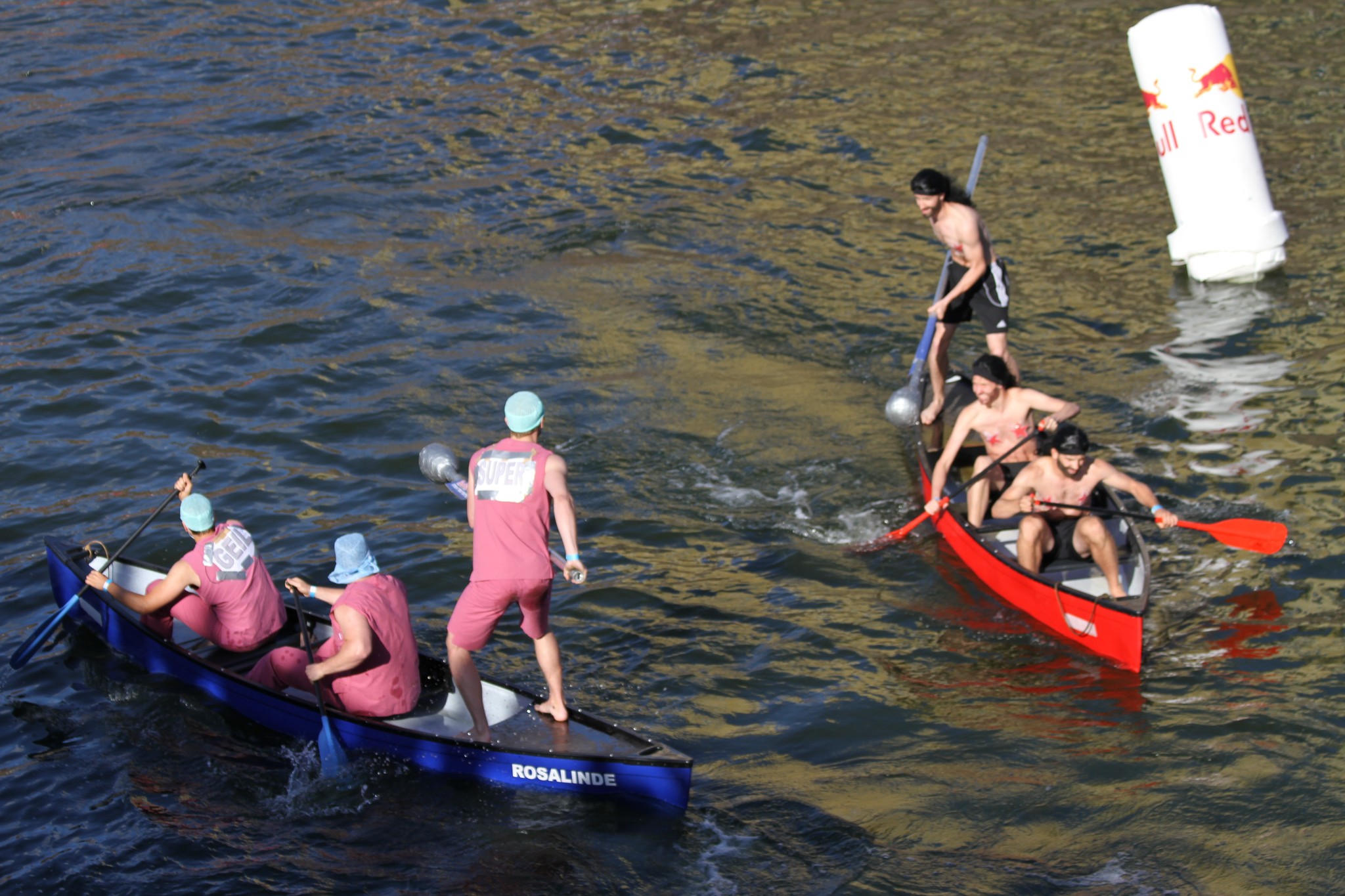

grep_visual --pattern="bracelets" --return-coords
[103,579,113,592]
[310,585,316,598]
[934,497,940,501]
[1150,505,1164,514]
[565,554,579,560]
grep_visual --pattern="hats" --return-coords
[1051,422,1089,455]
[910,168,948,196]
[972,354,1014,387]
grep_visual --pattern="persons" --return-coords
[247,533,421,717]
[925,353,1081,528]
[445,391,587,741]
[992,424,1179,597]
[910,167,1019,425]
[85,473,288,652]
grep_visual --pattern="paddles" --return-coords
[884,420,1046,538]
[1030,494,1288,554]
[9,459,207,670]
[287,582,351,780]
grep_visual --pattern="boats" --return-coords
[913,358,1151,676]
[43,533,693,817]
